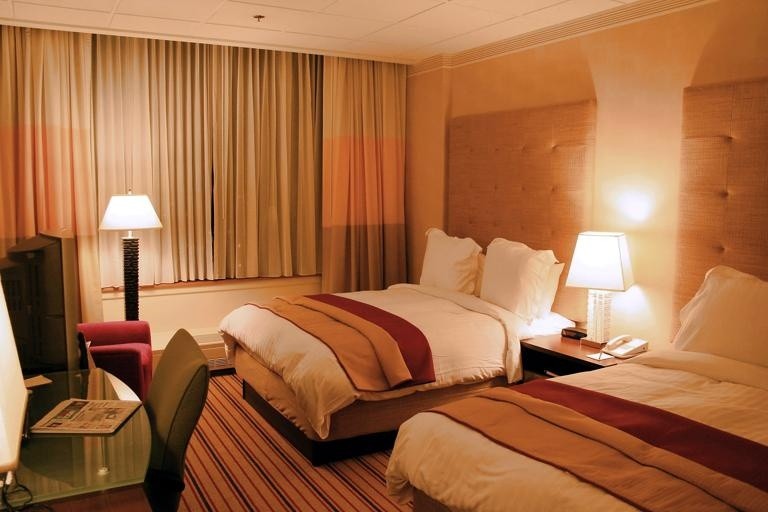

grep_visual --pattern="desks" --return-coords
[0,366,152,512]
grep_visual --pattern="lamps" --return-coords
[97,188,164,321]
[565,231,637,350]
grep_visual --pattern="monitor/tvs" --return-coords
[0,232,105,375]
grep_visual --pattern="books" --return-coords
[30,398,144,436]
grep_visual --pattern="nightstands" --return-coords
[520,336,635,378]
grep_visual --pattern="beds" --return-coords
[384,344,768,511]
[233,283,577,466]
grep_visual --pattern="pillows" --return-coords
[420,227,567,326]
[674,266,768,369]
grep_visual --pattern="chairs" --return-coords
[77,321,152,402]
[143,328,209,512]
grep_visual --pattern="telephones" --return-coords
[603,334,649,358]
